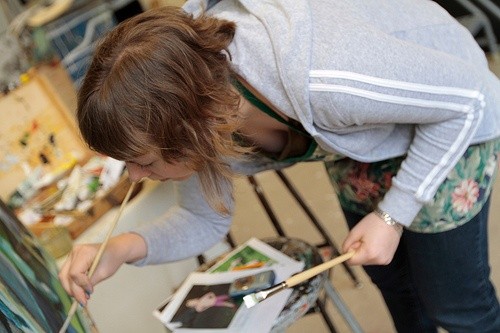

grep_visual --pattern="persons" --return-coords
[57,0,500,332]
[185,291,237,312]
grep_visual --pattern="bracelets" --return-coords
[374,206,403,236]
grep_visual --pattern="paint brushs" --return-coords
[54,180,139,333]
[242,250,355,308]
